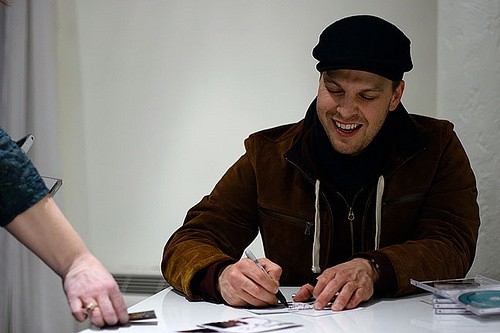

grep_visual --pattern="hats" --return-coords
[312,14,413,80]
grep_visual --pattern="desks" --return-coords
[79,286,500,333]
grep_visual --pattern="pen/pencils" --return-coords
[244,248,290,308]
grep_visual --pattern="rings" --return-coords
[87,302,96,311]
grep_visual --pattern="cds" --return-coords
[459,290,500,308]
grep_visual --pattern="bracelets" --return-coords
[370,256,381,279]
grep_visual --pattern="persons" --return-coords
[0,126,129,326]
[161,15,480,311]
